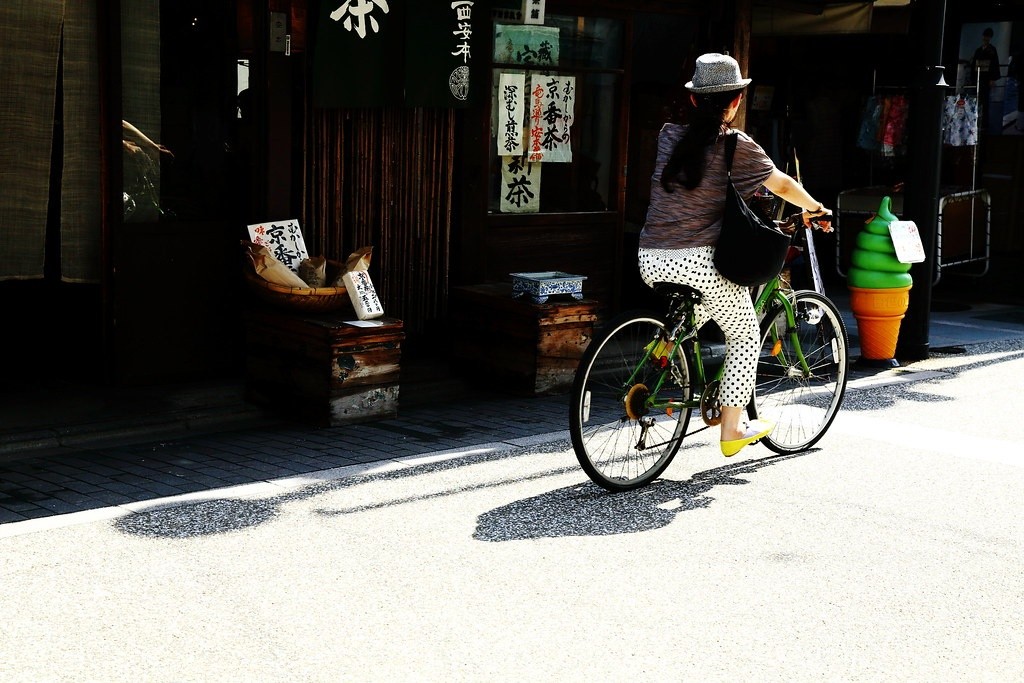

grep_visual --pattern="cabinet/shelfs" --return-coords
[263,315,406,429]
[457,283,598,398]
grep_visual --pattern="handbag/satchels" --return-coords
[714,128,792,286]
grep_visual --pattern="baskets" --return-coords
[243,260,350,314]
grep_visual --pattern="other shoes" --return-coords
[644,342,674,379]
[722,418,776,457]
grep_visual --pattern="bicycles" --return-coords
[567,196,848,495]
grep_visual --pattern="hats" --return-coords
[684,53,752,93]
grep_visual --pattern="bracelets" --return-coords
[806,203,824,215]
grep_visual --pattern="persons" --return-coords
[970,28,1001,88]
[638,53,833,457]
